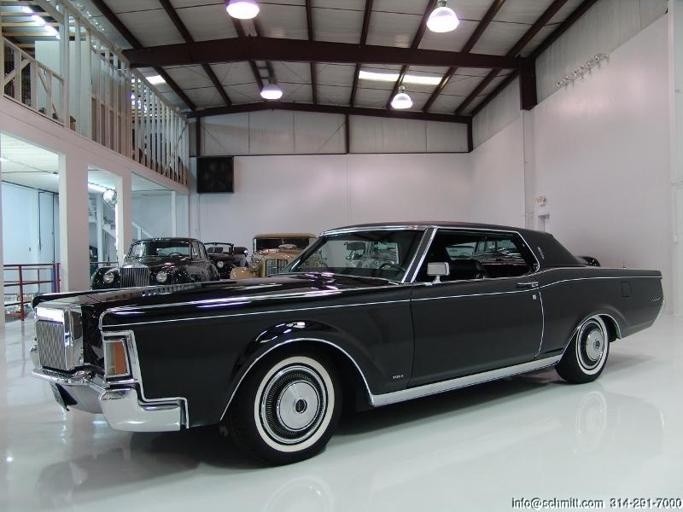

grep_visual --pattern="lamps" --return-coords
[259,82,284,100]
[224,0,261,20]
[389,85,413,110]
[426,1,460,35]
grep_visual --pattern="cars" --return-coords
[20,220,666,468]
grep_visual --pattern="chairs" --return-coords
[450,259,486,279]
